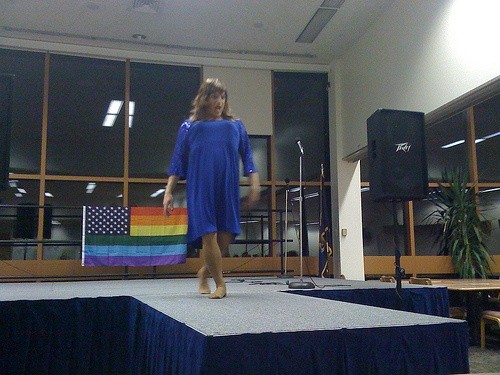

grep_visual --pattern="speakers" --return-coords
[0,73,15,189]
[367,108,429,200]
[15,204,53,239]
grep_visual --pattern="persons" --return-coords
[163,78,260,299]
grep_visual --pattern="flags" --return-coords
[319,174,333,278]
[81,206,190,265]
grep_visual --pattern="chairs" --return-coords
[380,276,396,283]
[409,277,469,319]
[480,310,500,351]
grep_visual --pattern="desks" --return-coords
[402,279,500,345]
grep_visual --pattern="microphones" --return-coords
[296,138,304,155]
[286,178,290,192]
